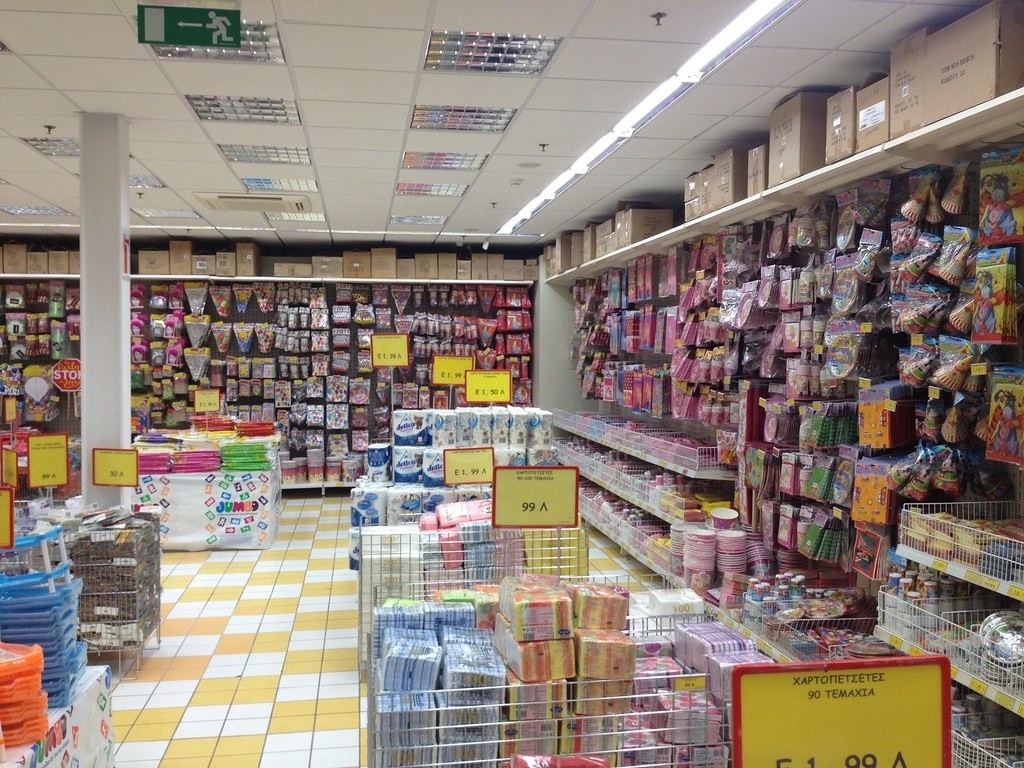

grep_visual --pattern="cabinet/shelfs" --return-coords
[872,499,1024,768]
[550,409,737,578]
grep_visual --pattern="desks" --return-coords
[0,665,114,768]
[135,453,280,551]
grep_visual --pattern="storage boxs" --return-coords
[0,0,1024,280]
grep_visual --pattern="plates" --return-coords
[764,587,878,642]
[981,611,1024,685]
[848,644,895,659]
[956,726,1024,768]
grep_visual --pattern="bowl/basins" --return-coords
[670,508,808,594]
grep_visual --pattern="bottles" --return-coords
[743,573,807,629]
